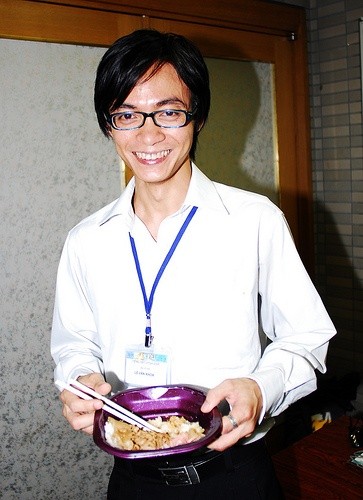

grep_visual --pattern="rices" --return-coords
[104,415,206,450]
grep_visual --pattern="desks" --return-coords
[268,414,363,500]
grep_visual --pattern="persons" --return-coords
[48,28,336,500]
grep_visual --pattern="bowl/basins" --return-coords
[92,384,222,458]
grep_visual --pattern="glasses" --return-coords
[105,107,198,130]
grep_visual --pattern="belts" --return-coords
[127,452,230,489]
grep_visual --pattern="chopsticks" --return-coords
[54,377,159,433]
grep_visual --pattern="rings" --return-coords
[227,414,240,431]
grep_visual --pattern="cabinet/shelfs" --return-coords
[0,0,317,499]
